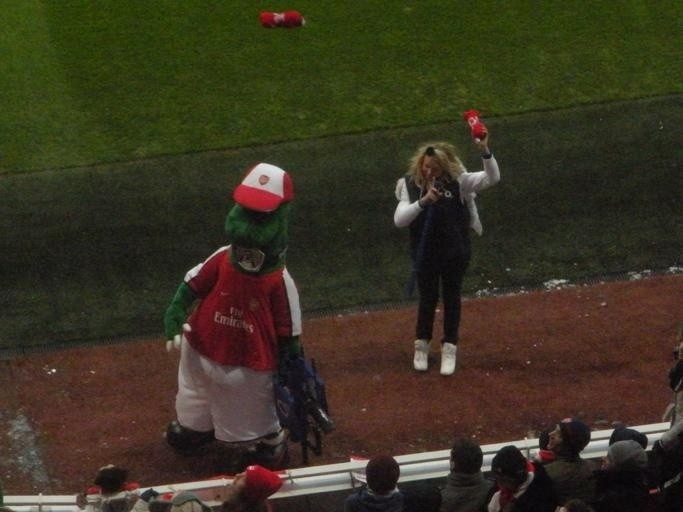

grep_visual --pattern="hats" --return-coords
[492,446,527,483]
[366,453,400,483]
[247,465,283,498]
[608,429,648,471]
[451,440,482,469]
[168,491,213,512]
[560,417,577,441]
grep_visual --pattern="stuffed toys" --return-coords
[165,162,303,482]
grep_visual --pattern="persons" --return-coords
[393,127,502,377]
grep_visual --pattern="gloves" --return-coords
[140,488,158,502]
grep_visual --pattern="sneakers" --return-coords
[440,335,457,376]
[413,339,431,371]
